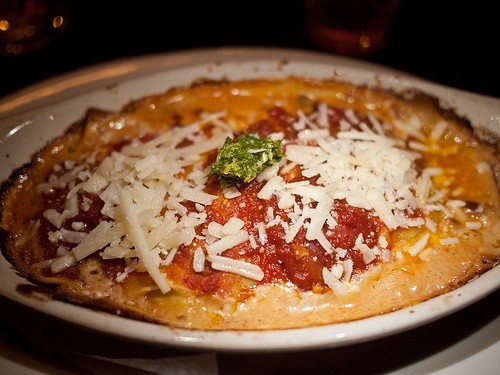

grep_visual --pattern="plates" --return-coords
[0,46,500,354]
